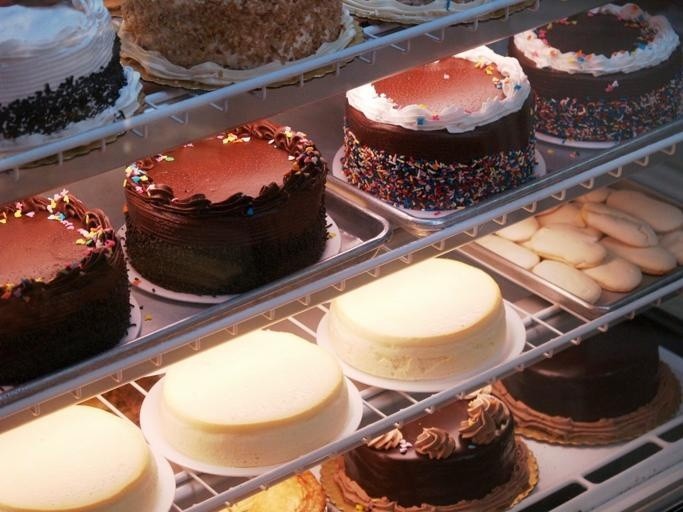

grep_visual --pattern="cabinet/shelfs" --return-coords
[0,1,682,509]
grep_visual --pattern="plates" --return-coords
[115,212,341,304]
[139,375,365,478]
[3,291,142,394]
[534,126,620,151]
[330,141,548,221]
[143,440,177,512]
[315,304,527,393]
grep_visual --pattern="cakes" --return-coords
[163,330,349,468]
[218,469,326,512]
[0,189,131,384]
[492,294,674,441]
[122,119,330,297]
[118,0,358,87]
[507,0,683,142]
[0,404,158,512]
[332,384,530,512]
[328,257,506,380]
[0,0,129,141]
[340,46,539,212]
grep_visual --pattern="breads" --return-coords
[475,186,683,305]
